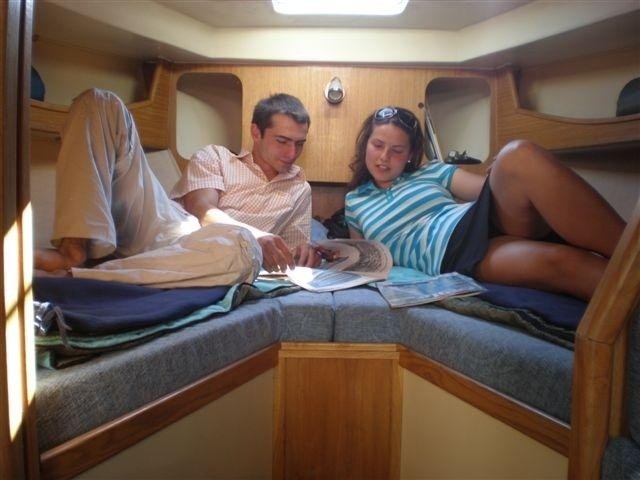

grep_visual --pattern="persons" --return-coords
[314,106,629,305]
[35,85,323,290]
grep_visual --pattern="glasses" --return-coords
[375,107,418,130]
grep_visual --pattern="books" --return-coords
[374,269,490,309]
[282,235,395,293]
